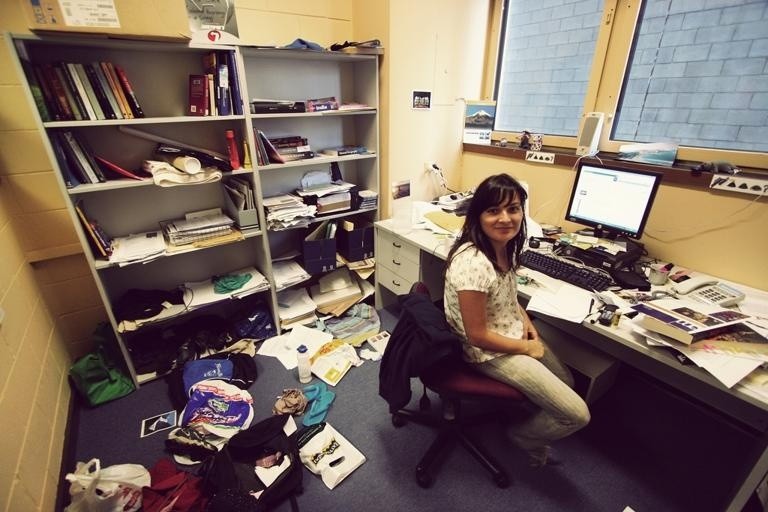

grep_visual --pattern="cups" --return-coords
[648,264,669,286]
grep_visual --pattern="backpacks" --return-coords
[167,351,257,410]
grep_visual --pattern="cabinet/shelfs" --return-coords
[5,28,279,391]
[239,45,380,334]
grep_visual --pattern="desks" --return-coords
[373,191,767,511]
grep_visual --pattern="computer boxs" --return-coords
[553,228,644,276]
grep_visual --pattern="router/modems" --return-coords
[616,269,653,291]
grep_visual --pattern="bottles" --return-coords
[296,345,313,384]
[172,156,201,174]
[225,129,241,170]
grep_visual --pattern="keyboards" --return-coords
[517,249,612,294]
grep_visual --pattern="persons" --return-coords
[439,174,594,474]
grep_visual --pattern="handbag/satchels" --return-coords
[67,352,136,408]
[199,412,304,512]
[63,457,153,512]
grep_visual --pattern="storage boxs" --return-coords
[334,215,376,262]
[289,230,336,270]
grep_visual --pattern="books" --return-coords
[249,96,377,114]
[46,129,252,189]
[279,270,376,328]
[263,180,378,232]
[74,174,256,268]
[252,126,367,168]
[460,98,498,148]
[630,293,751,345]
[188,50,243,118]
[14,38,144,123]
[271,220,376,292]
[364,329,394,359]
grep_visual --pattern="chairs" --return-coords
[394,279,566,490]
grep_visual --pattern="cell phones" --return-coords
[598,304,618,325]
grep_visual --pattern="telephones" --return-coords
[670,274,746,309]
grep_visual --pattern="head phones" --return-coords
[528,235,578,255]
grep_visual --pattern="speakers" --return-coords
[574,111,604,157]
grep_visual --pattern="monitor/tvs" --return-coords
[564,161,662,248]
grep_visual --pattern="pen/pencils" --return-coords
[589,297,594,315]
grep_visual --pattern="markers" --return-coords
[590,309,605,325]
[660,262,674,274]
